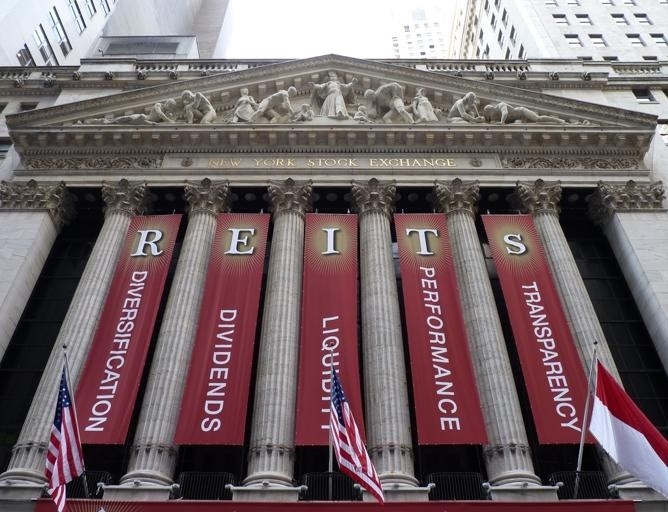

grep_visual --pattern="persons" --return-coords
[355,82,565,124]
[308,72,357,118]
[112,85,313,124]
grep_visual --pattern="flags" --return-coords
[43,366,87,512]
[591,357,668,495]
[328,366,385,506]
